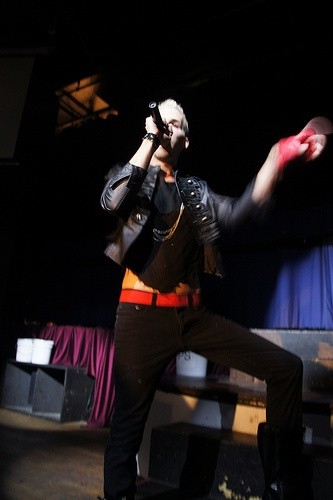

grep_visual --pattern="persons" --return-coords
[98,100,329,499]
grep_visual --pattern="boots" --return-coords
[257,422,317,500]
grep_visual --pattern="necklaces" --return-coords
[152,201,184,243]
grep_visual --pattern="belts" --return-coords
[119,288,200,307]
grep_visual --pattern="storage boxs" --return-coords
[16,338,54,365]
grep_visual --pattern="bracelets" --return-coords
[143,133,160,147]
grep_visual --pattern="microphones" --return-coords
[149,102,166,134]
